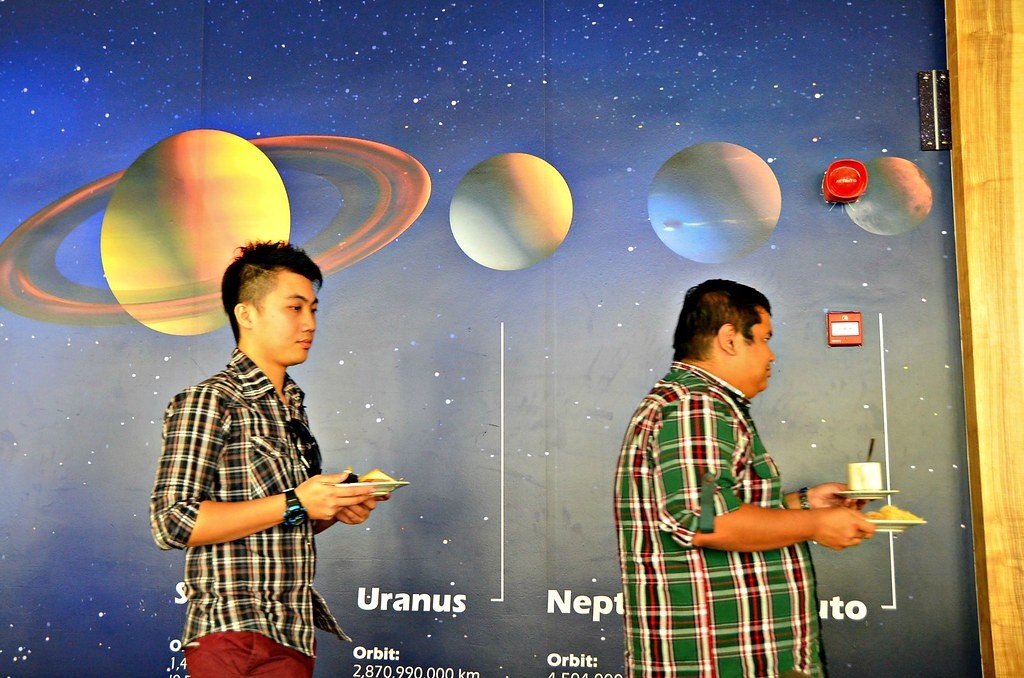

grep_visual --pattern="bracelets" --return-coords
[798,486,810,511]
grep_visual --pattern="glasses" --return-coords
[286,418,321,476]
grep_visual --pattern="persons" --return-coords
[147,243,391,678]
[613,281,876,678]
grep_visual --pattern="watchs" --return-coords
[284,488,308,526]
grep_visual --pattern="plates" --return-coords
[834,490,902,500]
[862,519,927,533]
[336,481,409,495]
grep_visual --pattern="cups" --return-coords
[848,462,881,492]
